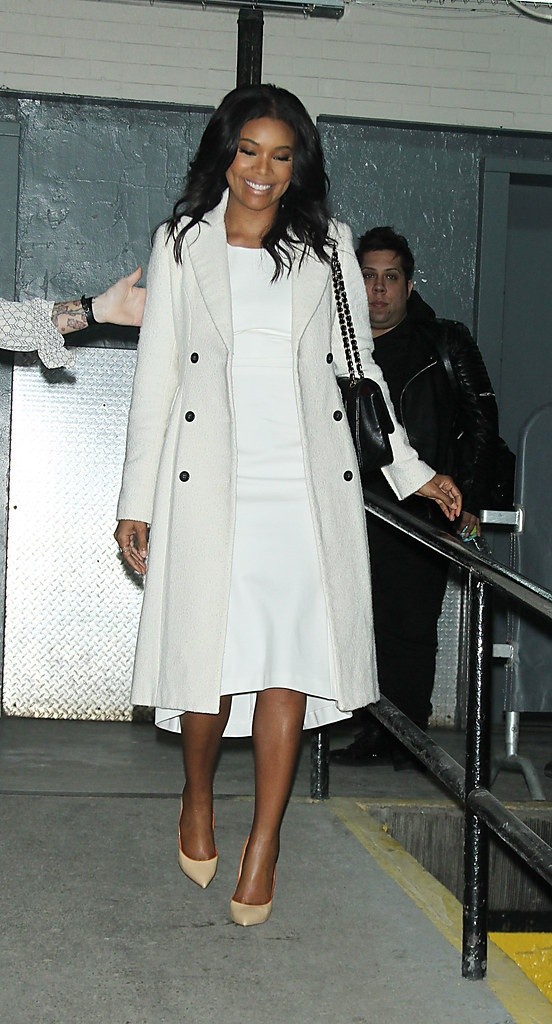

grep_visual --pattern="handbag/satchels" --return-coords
[457,431,516,510]
[337,376,395,472]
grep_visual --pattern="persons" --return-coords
[0,266,147,370]
[110,80,462,931]
[326,223,517,771]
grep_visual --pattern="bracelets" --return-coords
[80,294,98,326]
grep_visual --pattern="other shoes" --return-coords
[544,760,552,778]
[178,796,219,889]
[329,731,427,772]
[230,835,276,926]
